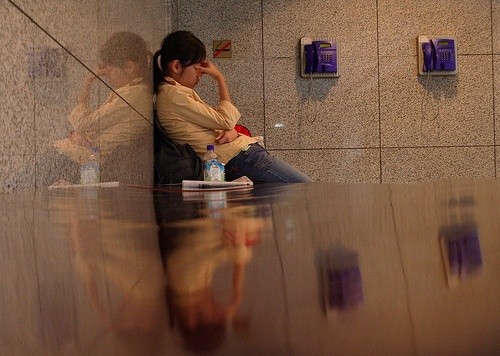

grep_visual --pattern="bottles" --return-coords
[203,145,218,181]
[80,147,100,184]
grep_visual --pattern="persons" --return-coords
[153,186,286,356]
[152,30,315,185]
[13,30,154,185]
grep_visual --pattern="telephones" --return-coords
[304,41,338,74]
[422,39,456,72]
